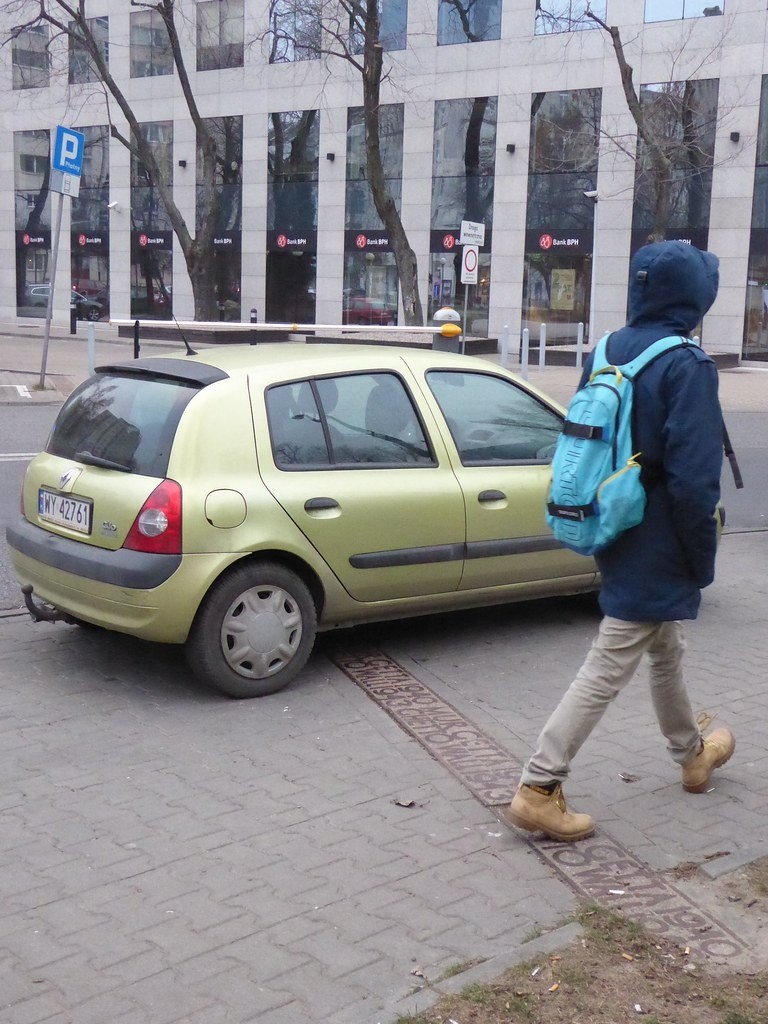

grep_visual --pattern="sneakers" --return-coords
[681,727,735,793]
[504,782,595,842]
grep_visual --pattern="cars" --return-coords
[6,342,724,698]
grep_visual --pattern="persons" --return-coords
[506,241,745,842]
[533,270,544,302]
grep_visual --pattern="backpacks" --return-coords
[545,331,744,557]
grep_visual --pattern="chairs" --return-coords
[290,379,346,454]
[355,384,416,465]
